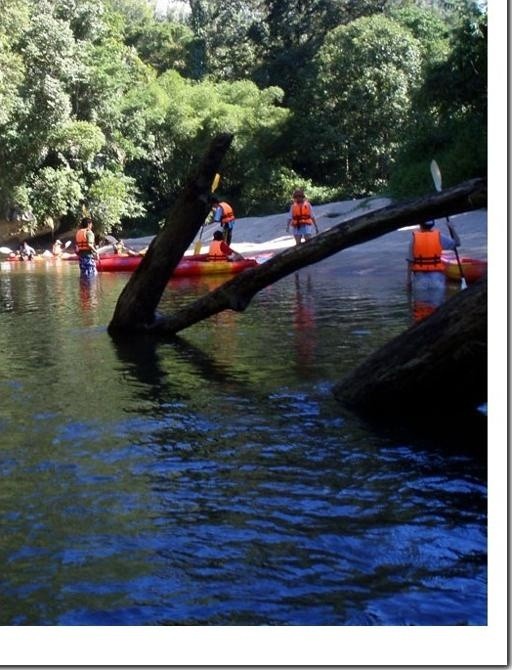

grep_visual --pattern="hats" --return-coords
[293,190,305,198]
[420,219,435,230]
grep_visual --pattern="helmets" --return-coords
[211,194,219,203]
[213,231,223,239]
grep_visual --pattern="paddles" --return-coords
[431,161,467,291]
[194,173,219,254]
[105,235,144,256]
[61,241,71,252]
[0,247,14,254]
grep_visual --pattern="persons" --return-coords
[292,268,321,372]
[115,239,125,255]
[286,189,319,245]
[20,243,41,258]
[208,231,244,263]
[409,218,463,270]
[210,194,236,248]
[78,270,103,306]
[53,240,66,256]
[76,217,98,270]
[407,271,444,325]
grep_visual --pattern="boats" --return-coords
[440,255,487,284]
[90,252,262,276]
